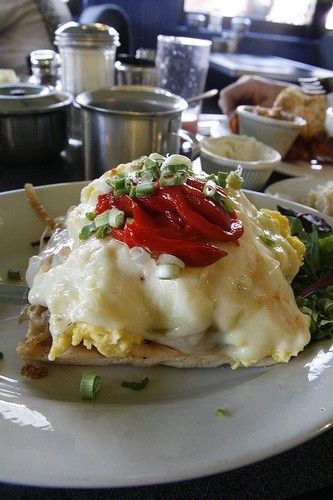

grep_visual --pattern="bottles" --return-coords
[54,21,121,141]
[26,49,62,92]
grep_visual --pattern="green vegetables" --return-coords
[275,204,333,344]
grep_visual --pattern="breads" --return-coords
[272,86,328,138]
[17,304,281,368]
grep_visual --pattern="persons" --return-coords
[0,0,74,78]
[217,73,333,121]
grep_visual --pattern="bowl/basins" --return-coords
[237,105,306,162]
[200,136,282,192]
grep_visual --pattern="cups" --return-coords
[0,83,74,190]
[75,85,187,179]
[155,35,213,137]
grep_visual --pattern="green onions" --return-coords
[79,151,277,417]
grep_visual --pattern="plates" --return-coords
[0,180,333,487]
[200,114,333,178]
[264,177,333,213]
